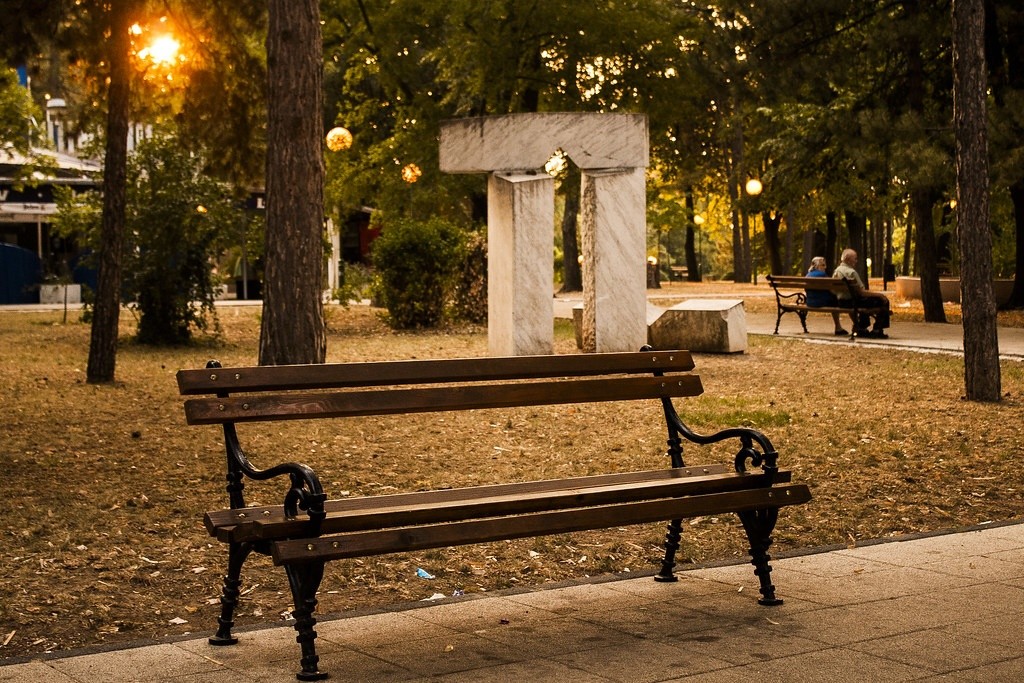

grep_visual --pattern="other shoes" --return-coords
[857,330,889,338]
[835,330,849,335]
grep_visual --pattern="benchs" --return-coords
[176,344,814,683]
[766,273,893,342]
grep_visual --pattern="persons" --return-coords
[805,256,849,336]
[831,248,892,341]
